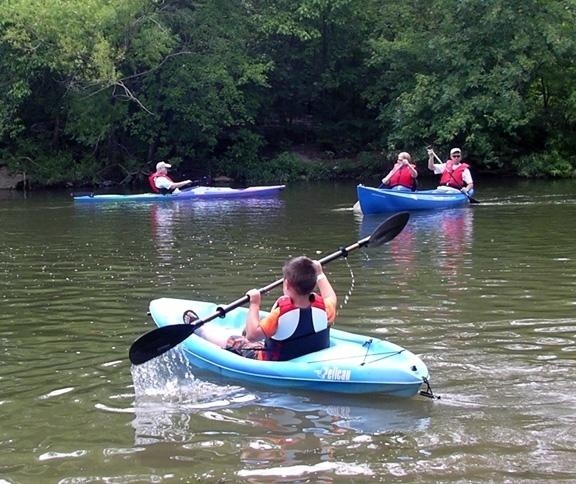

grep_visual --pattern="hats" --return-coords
[156,161,172,169]
[450,148,461,154]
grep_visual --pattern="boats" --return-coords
[357,184,474,215]
[71,185,286,203]
[147,298,440,395]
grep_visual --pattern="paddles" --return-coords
[189,176,233,184]
[129,211,410,366]
[353,161,404,211]
[425,145,481,204]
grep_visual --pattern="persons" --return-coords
[381,152,418,191]
[148,161,192,195]
[151,204,175,262]
[181,255,338,362]
[438,206,473,271]
[390,215,417,265]
[236,409,345,483]
[427,147,474,194]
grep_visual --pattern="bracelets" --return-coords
[317,273,325,282]
